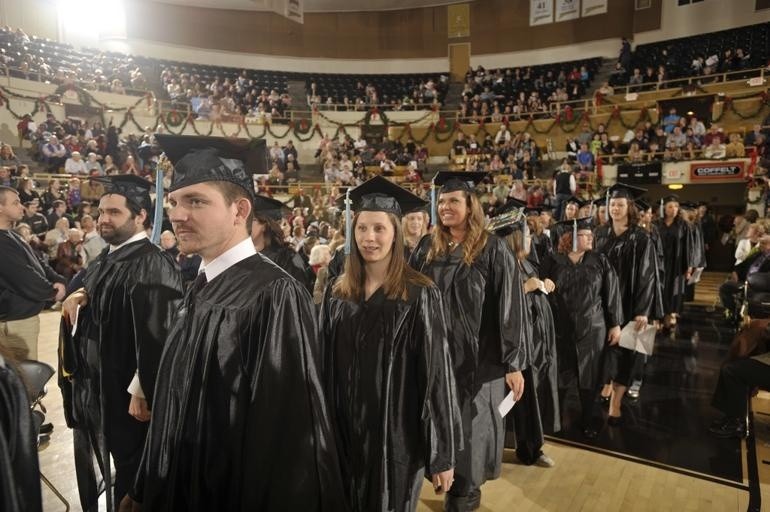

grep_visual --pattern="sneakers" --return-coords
[535,454,554,467]
[443,488,482,512]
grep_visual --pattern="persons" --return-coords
[0,25,770,512]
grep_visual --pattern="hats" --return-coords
[335,170,491,256]
[78,133,293,245]
[485,182,649,253]
[659,193,712,219]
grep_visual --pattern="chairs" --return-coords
[1,30,594,112]
[632,20,770,92]
